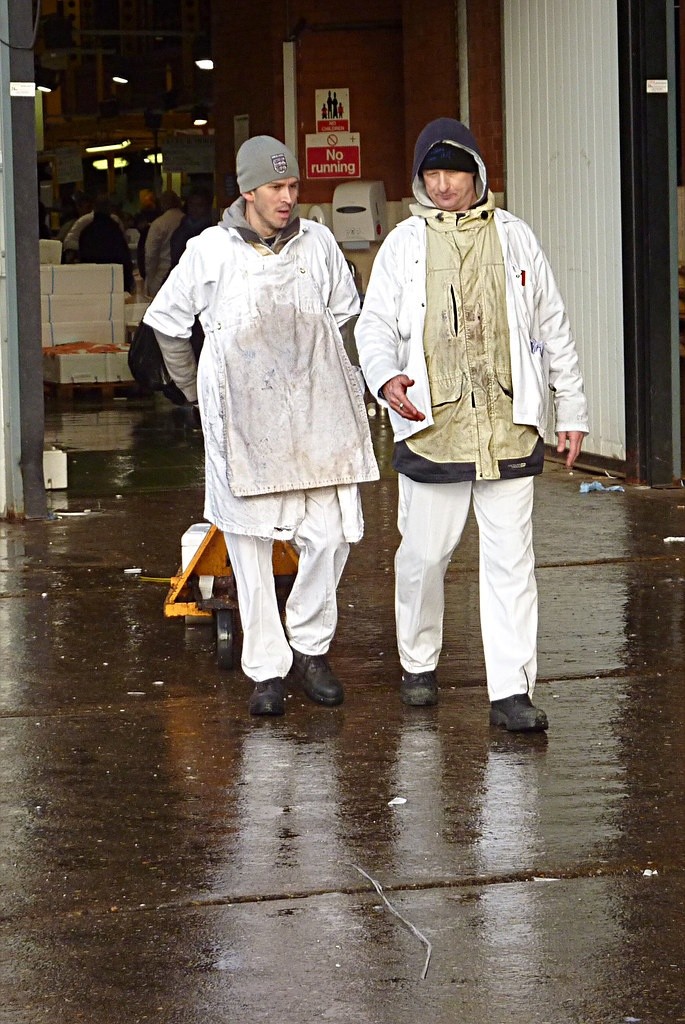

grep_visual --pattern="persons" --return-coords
[354,117,589,734]
[141,135,362,718]
[35,187,216,298]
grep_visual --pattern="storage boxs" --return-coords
[44,445,68,489]
[40,235,152,384]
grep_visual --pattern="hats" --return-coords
[235,136,300,193]
[420,144,478,173]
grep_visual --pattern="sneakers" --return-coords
[490,694,550,731]
[401,670,439,706]
[251,677,287,715]
[292,650,344,704]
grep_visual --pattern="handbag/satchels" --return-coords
[128,318,172,392]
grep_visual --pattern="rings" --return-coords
[400,403,404,408]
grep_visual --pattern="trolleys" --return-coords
[163,523,301,672]
